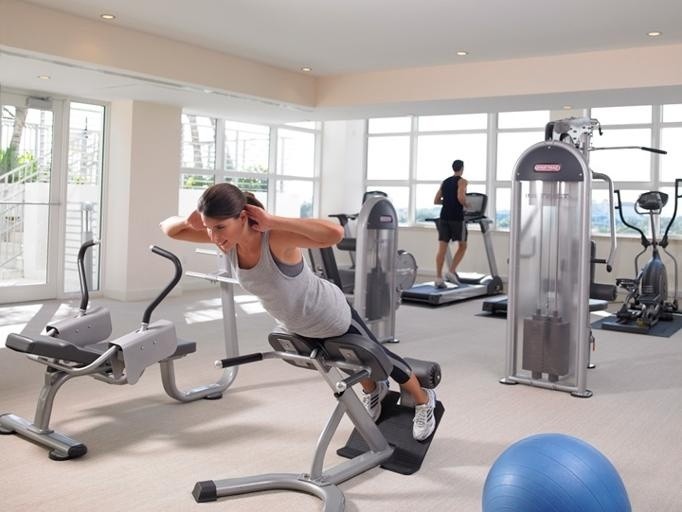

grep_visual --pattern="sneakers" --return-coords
[362,379,389,422]
[435,278,447,288]
[446,272,461,285]
[412,388,436,441]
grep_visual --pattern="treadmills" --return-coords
[401,192,508,308]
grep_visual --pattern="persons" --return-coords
[433,160,474,290]
[161,183,439,442]
